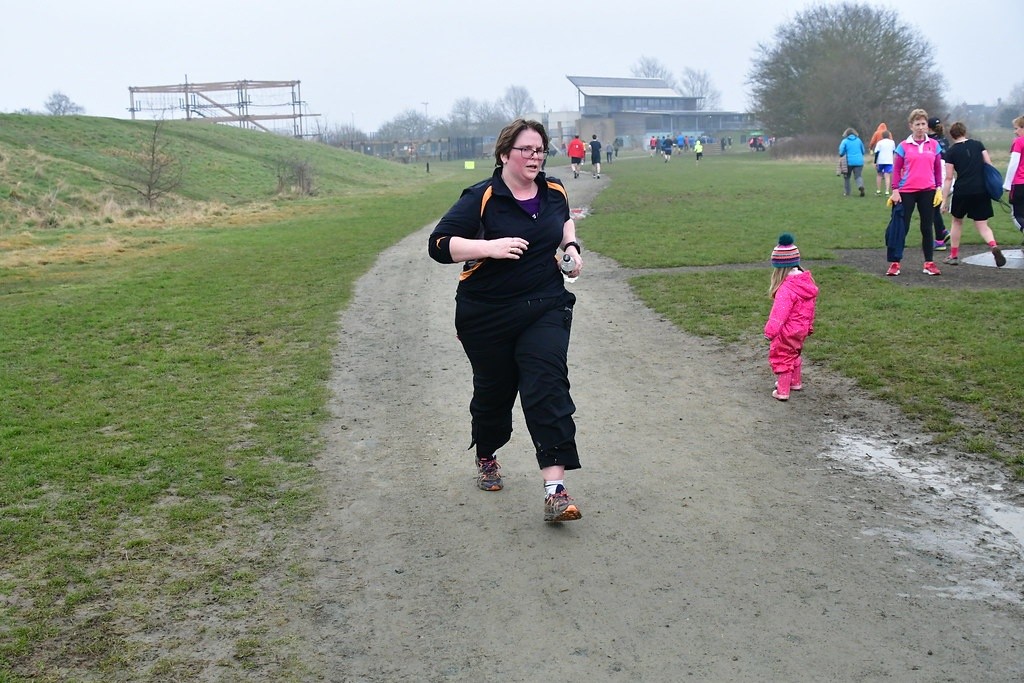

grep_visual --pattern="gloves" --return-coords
[932,188,942,208]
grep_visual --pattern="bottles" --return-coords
[561,254,578,283]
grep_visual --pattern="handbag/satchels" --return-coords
[983,163,1003,202]
[887,199,892,208]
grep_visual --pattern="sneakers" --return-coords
[933,240,946,250]
[923,262,941,276]
[942,230,950,243]
[943,256,959,265]
[886,262,901,275]
[544,484,582,521]
[992,246,1006,267]
[474,455,503,490]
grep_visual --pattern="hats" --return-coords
[928,117,941,129]
[771,234,800,267]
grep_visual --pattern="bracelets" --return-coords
[564,241,581,254]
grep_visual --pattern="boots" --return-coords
[775,367,802,390]
[772,373,792,400]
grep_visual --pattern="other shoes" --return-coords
[844,193,851,196]
[885,194,891,197]
[874,191,881,196]
[859,186,865,197]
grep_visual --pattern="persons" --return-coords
[1003,114,1024,253]
[606,136,623,164]
[567,133,584,178]
[650,132,735,163]
[693,140,704,162]
[728,136,731,145]
[939,121,1007,267]
[928,116,950,251]
[749,135,765,151]
[870,122,893,179]
[874,131,896,195]
[582,141,587,163]
[885,107,943,275]
[764,233,819,400]
[720,137,725,149]
[428,119,585,522]
[839,128,866,197]
[588,135,602,179]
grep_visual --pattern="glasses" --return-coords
[511,147,549,160]
